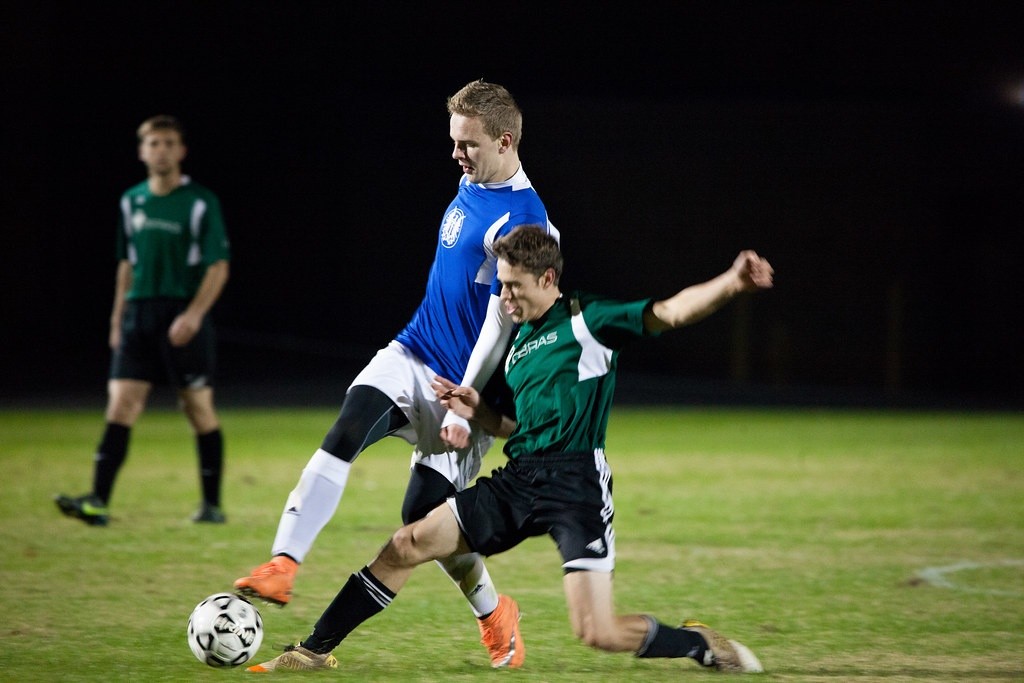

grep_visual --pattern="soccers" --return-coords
[186,592,262,668]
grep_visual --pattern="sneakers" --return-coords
[677,619,764,675]
[477,593,525,670]
[193,505,226,526]
[235,556,299,609]
[55,493,110,526]
[244,642,338,674]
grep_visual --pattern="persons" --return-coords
[55,118,229,526]
[247,227,775,676]
[235,79,564,672]
